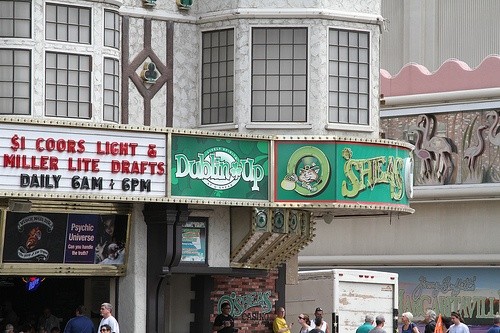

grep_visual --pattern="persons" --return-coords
[0,302,119,333]
[215,303,500,333]
[94,215,125,263]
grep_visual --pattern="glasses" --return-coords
[100,329,107,332]
[298,316,306,320]
